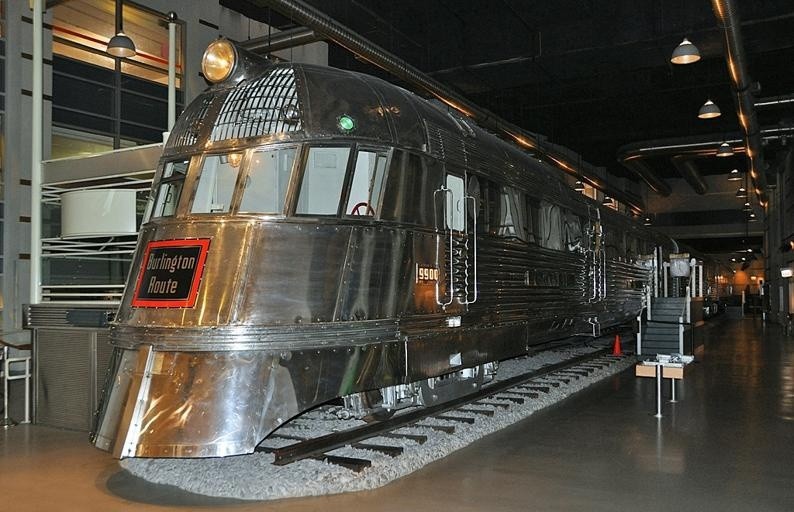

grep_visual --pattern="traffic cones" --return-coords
[612,336,623,355]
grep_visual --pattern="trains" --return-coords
[91,40,735,459]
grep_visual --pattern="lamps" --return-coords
[200,39,271,86]
[670,35,758,222]
[106,1,136,59]
